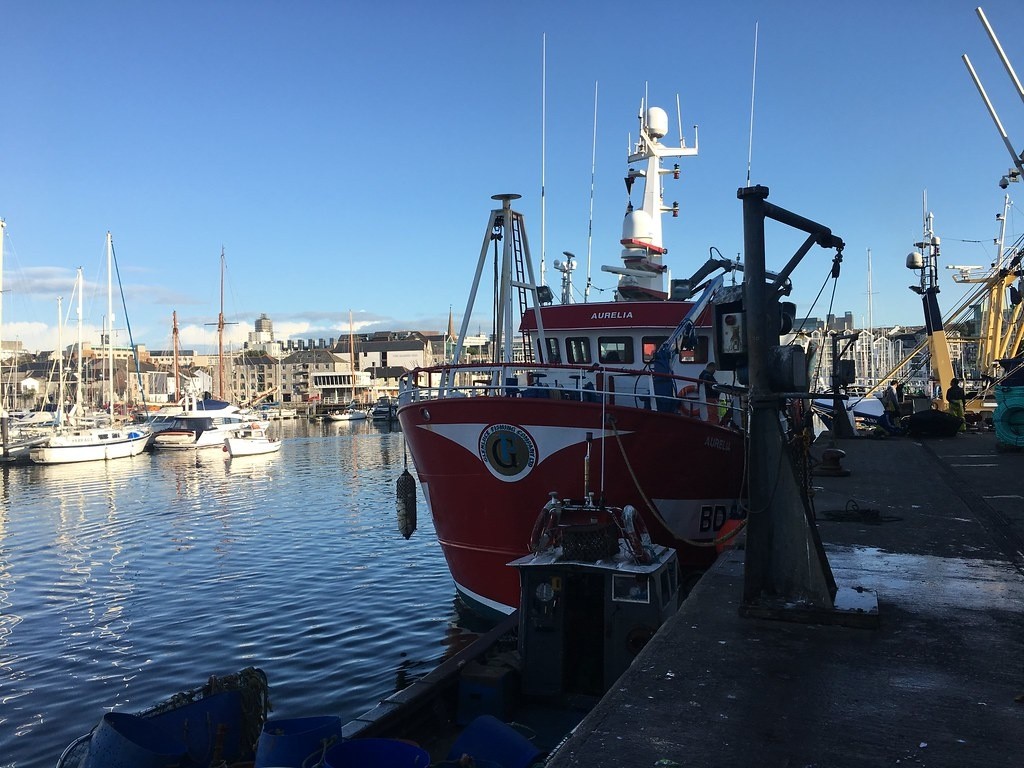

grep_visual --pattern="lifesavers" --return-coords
[529,496,562,555]
[621,506,657,565]
[677,385,700,416]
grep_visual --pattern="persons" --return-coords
[697,362,720,425]
[887,380,903,425]
[946,378,966,432]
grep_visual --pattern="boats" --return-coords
[811,384,883,431]
[224,423,281,456]
[372,395,399,420]
[373,17,804,641]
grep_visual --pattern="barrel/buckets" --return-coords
[324,739,430,768]
[255,716,341,768]
[450,714,542,768]
[88,712,190,768]
[162,690,261,763]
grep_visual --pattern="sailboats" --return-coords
[0,217,184,463]
[140,244,272,449]
[323,309,367,420]
